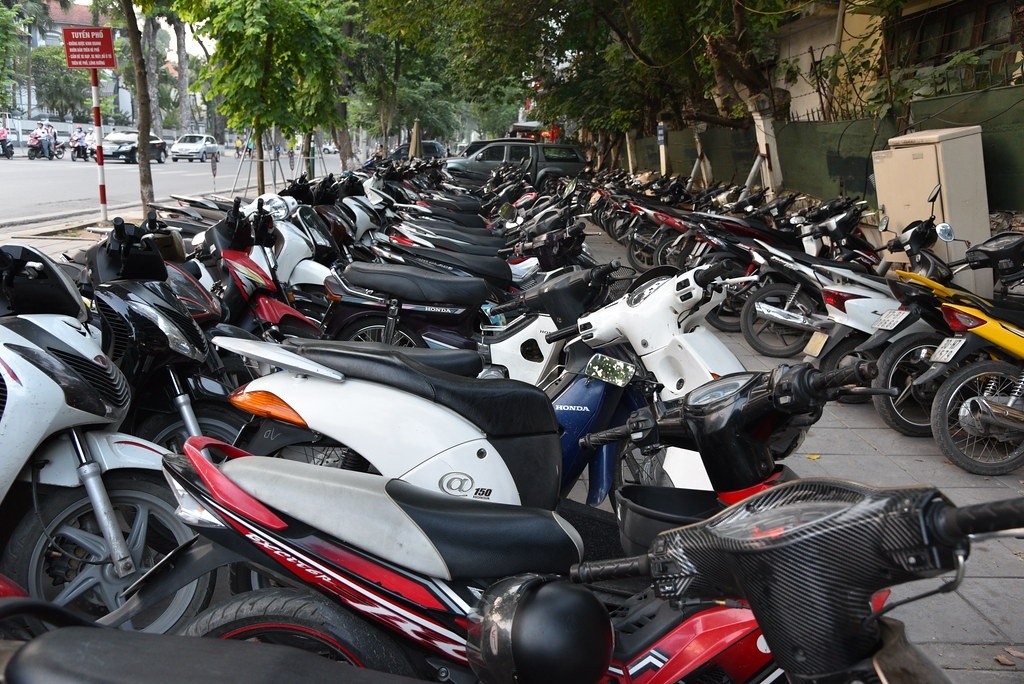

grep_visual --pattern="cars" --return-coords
[382,141,444,160]
[456,137,535,160]
[170,134,221,162]
[322,145,338,153]
[100,130,167,164]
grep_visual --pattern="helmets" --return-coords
[88,127,93,131]
[37,121,43,126]
[43,121,50,126]
[76,124,82,128]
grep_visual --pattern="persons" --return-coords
[235,136,281,160]
[446,148,451,158]
[374,144,384,161]
[0,120,116,162]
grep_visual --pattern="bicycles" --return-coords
[234,145,257,161]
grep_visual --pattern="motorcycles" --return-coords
[53,139,66,159]
[26,129,54,160]
[0,138,14,159]
[69,136,89,161]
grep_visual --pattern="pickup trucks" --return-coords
[439,141,587,197]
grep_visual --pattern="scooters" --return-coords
[0,156,1023,684]
